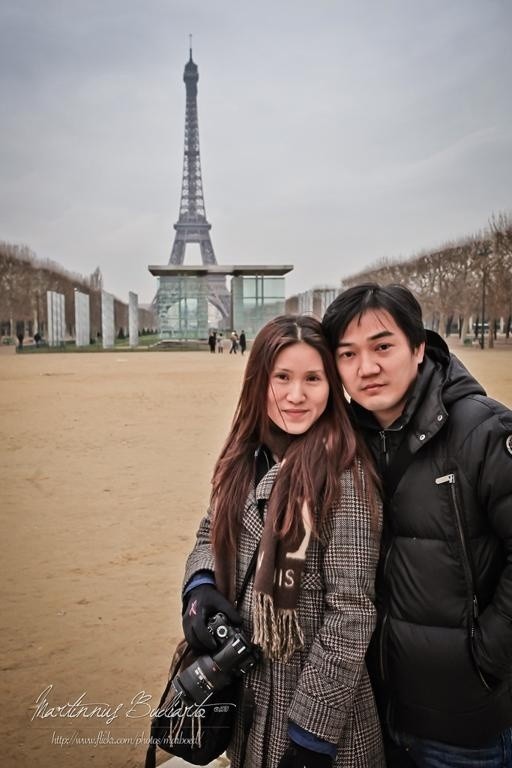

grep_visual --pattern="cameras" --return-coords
[170,611,261,711]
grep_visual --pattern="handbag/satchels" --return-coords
[144,639,255,768]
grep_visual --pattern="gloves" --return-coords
[278,741,331,768]
[182,584,245,650]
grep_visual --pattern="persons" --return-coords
[181,282,512,768]
[17,333,41,352]
